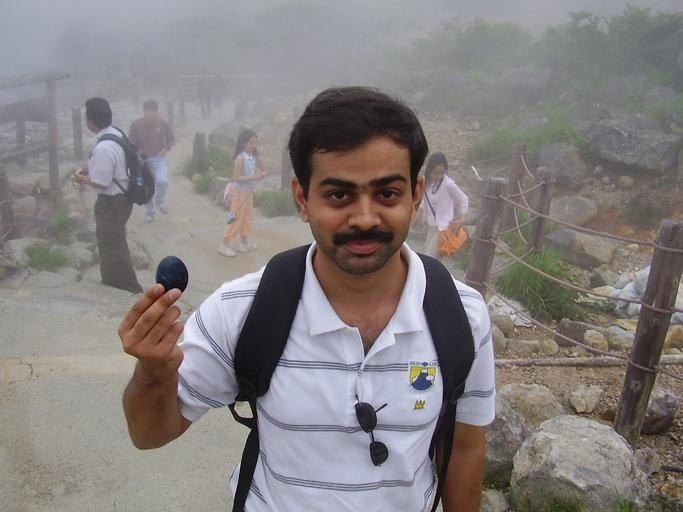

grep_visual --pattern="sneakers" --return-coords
[240,243,257,252]
[146,204,167,221]
[219,247,236,257]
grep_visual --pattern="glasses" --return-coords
[355,394,388,467]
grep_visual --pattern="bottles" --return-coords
[134,174,144,192]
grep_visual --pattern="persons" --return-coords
[116,88,496,512]
[419,152,469,259]
[129,100,174,221]
[217,127,263,257]
[72,97,144,293]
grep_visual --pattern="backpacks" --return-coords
[97,127,155,206]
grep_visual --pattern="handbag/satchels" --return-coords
[438,224,466,255]
[223,183,232,208]
